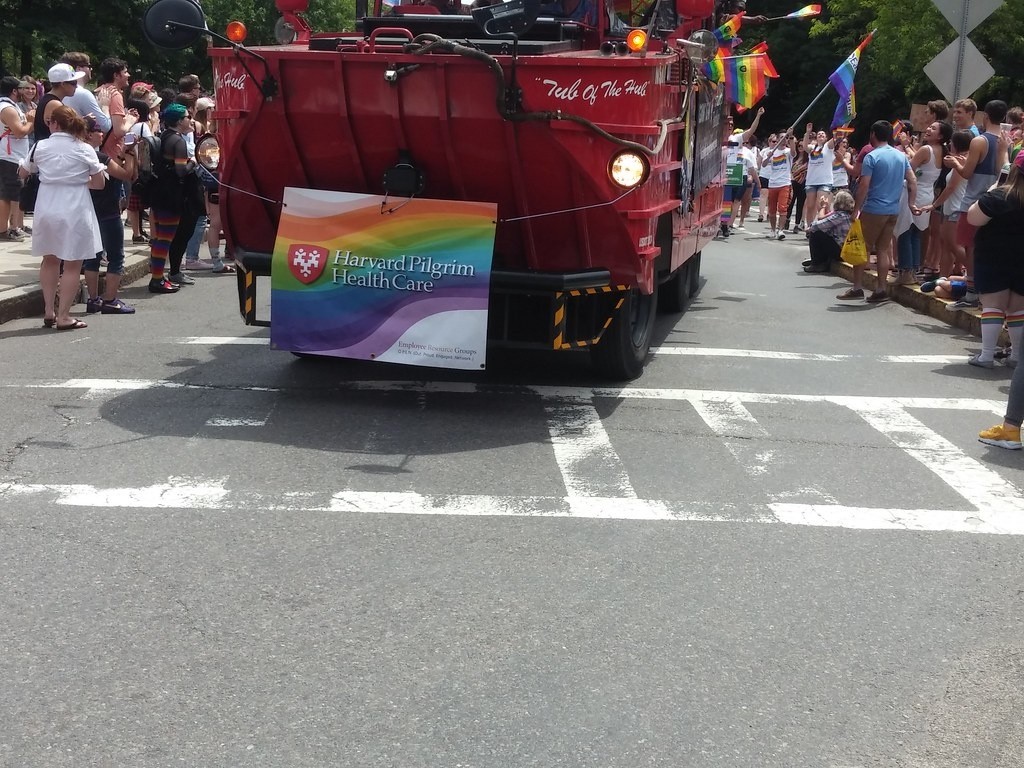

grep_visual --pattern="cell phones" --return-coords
[124,132,134,145]
[997,169,1010,188]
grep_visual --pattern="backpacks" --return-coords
[138,131,176,178]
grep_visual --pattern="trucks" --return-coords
[142,1,740,383]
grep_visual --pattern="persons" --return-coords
[721,98,1024,450]
[715,0,767,33]
[0,51,236,332]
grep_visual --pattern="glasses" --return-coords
[182,114,192,120]
[64,81,77,86]
[742,136,803,148]
[47,118,53,125]
[90,128,101,132]
[81,63,91,68]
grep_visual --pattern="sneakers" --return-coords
[978,424,1022,450]
[1,210,238,330]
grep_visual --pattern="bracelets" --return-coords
[932,202,936,209]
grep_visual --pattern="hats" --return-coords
[48,63,86,83]
[196,97,215,111]
[733,128,744,134]
[129,81,162,109]
[1014,150,1024,174]
[163,104,186,125]
[0,77,28,96]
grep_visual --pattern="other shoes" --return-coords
[835,260,982,312]
[802,259,831,272]
[968,354,993,368]
[718,213,813,240]
[994,346,1020,369]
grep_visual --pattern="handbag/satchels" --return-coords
[840,217,867,265]
[19,140,40,211]
